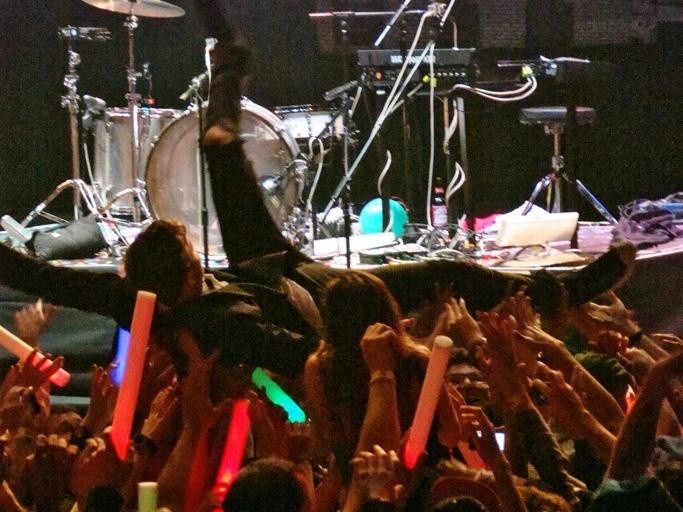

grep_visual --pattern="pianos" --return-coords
[354,46,594,87]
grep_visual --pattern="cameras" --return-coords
[475,428,506,453]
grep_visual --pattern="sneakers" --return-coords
[195,0,249,47]
[564,239,637,309]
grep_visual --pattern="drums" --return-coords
[143,96,308,271]
[82,103,182,225]
[269,100,356,143]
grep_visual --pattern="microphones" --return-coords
[260,175,282,200]
[324,80,359,100]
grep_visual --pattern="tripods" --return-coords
[10,66,155,251]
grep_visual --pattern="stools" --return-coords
[518,104,618,228]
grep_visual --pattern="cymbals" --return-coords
[82,0,188,20]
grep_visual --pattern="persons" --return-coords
[1,264,683,512]
[0,0,637,374]
